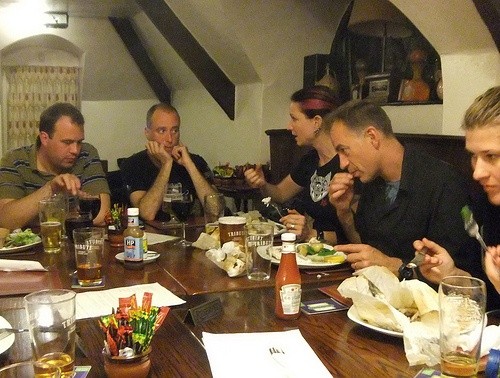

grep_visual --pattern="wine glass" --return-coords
[76,189,101,221]
[65,211,93,276]
[163,182,182,226]
[49,191,70,240]
[170,192,195,247]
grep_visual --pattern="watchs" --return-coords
[396,258,413,282]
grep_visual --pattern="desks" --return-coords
[213,178,259,214]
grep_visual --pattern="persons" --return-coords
[325,98,492,289]
[0,103,111,230]
[240,85,361,244]
[413,86,500,293]
[119,103,225,221]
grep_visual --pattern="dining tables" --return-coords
[0,208,500,378]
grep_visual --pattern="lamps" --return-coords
[346,0,415,73]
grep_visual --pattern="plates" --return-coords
[107,225,145,231]
[257,243,347,268]
[0,316,15,355]
[114,250,160,265]
[347,301,488,338]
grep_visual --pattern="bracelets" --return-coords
[316,229,324,239]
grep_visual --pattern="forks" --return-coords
[463,211,496,264]
[265,199,288,219]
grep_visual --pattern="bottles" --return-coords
[274,232,302,320]
[123,207,144,268]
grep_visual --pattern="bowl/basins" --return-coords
[0,231,40,254]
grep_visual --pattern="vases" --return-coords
[318,61,340,94]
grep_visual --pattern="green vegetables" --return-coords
[3,228,40,247]
[305,245,338,255]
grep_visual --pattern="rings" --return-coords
[290,223,295,229]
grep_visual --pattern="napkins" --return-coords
[0,258,49,272]
[201,328,334,378]
[35,282,186,328]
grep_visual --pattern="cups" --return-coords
[439,275,486,378]
[24,288,78,378]
[220,217,245,252]
[73,226,106,287]
[1,360,62,378]
[39,199,64,253]
[245,220,286,236]
[246,222,274,280]
[203,193,224,237]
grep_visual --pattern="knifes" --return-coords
[406,246,430,269]
